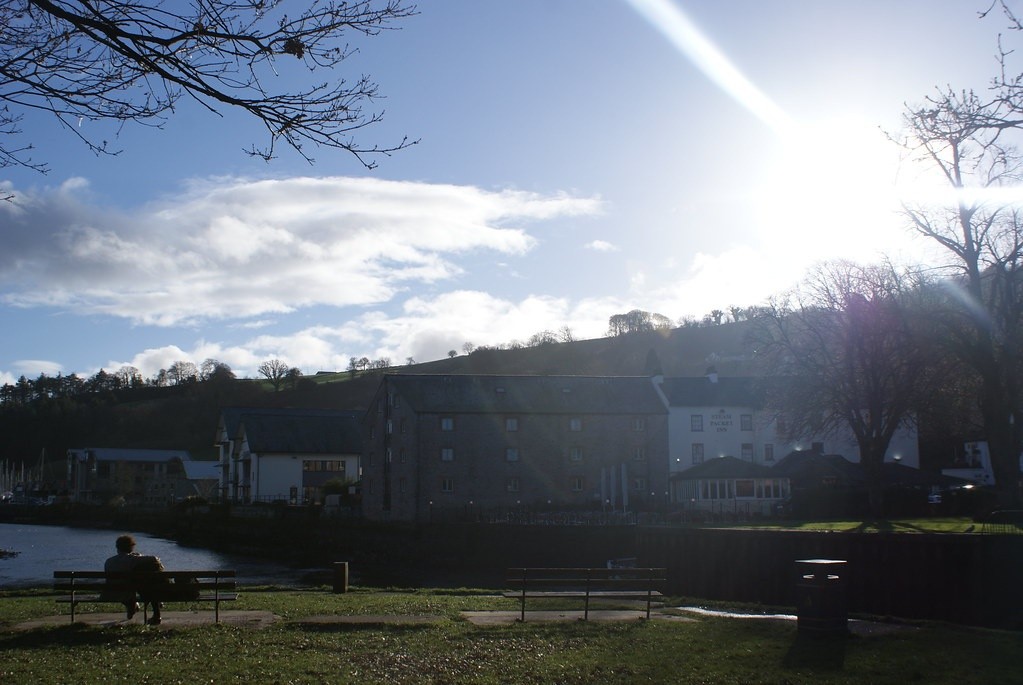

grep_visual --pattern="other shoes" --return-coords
[127,601,139,619]
[146,611,161,624]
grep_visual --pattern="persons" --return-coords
[104,535,179,625]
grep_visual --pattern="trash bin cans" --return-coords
[794,558,848,632]
[333,562,348,595]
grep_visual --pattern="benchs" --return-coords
[504,566,669,622]
[52,570,239,623]
